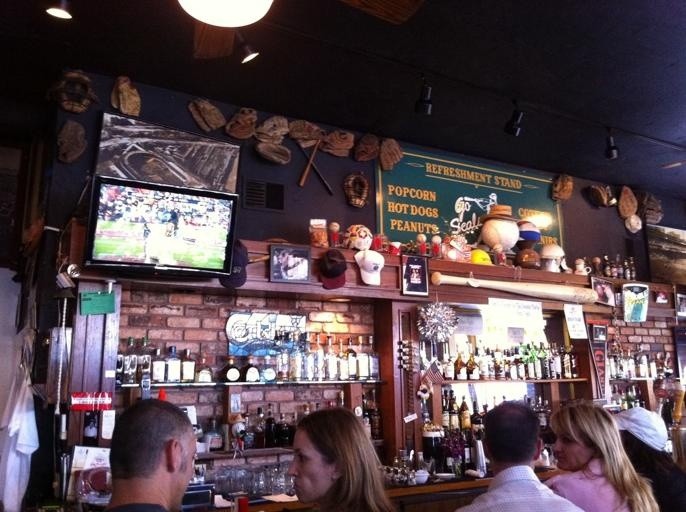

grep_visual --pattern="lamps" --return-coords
[43,0,73,19]
[603,128,620,161]
[505,106,525,138]
[233,26,260,65]
[416,77,434,114]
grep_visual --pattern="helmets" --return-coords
[540,244,565,259]
[470,249,493,265]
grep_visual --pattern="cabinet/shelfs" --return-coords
[69,275,395,500]
[609,378,679,413]
[416,301,589,460]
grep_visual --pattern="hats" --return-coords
[219,240,248,287]
[317,249,346,290]
[355,250,385,286]
[480,205,517,222]
[612,407,668,452]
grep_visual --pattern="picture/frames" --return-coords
[592,324,607,341]
[400,254,429,297]
[269,244,311,285]
[590,276,616,308]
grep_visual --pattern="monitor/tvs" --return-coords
[84,175,240,278]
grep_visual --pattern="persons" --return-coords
[98,184,233,262]
[542,403,660,511]
[453,400,585,511]
[103,399,196,512]
[286,407,400,512]
[612,406,686,512]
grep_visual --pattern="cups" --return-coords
[209,460,294,495]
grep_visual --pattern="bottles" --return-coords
[592,252,637,281]
[380,449,429,489]
[419,397,430,424]
[203,389,381,452]
[418,340,579,382]
[574,263,591,276]
[441,389,550,432]
[607,344,662,381]
[442,432,470,472]
[83,419,98,448]
[653,372,685,431]
[611,383,646,414]
[116,328,381,383]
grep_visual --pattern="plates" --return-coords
[435,473,455,481]
